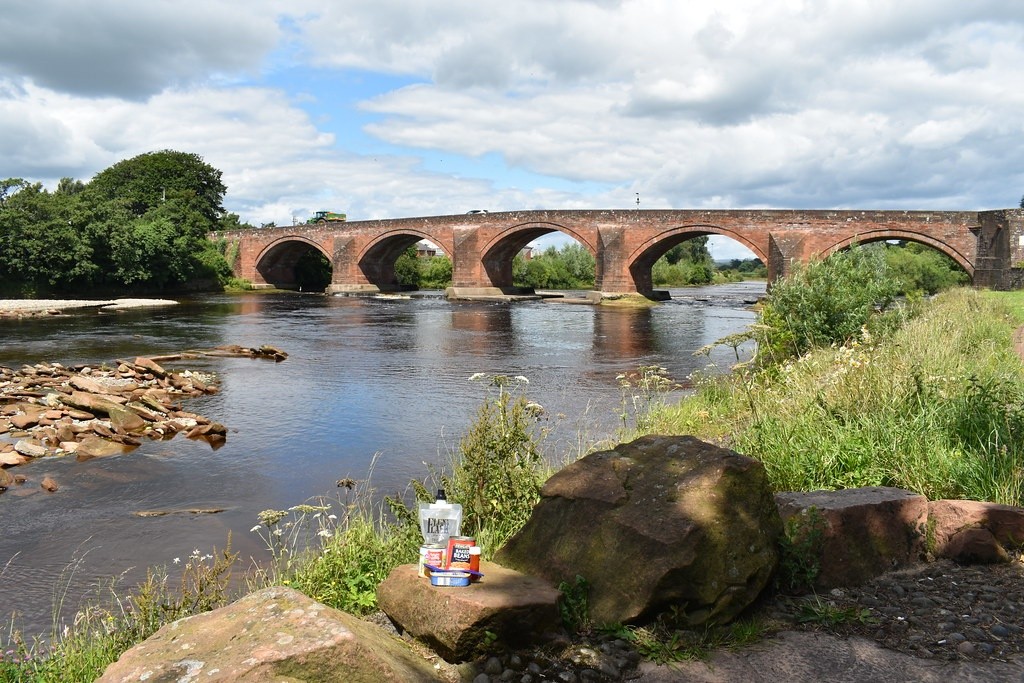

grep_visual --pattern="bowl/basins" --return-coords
[430,571,471,586]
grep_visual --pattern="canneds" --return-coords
[445,535,476,572]
[418,543,447,578]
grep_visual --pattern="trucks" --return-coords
[306,210,347,224]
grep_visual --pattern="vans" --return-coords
[466,209,489,215]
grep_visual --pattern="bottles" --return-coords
[469,546,481,583]
